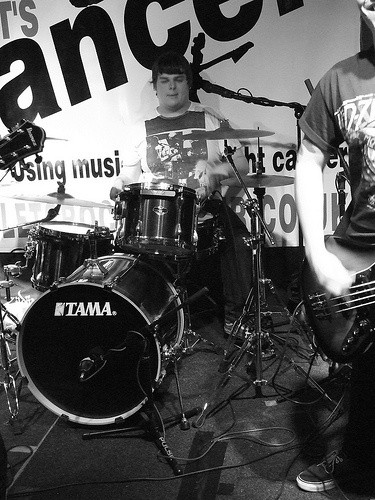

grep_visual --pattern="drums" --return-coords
[115,180,201,259]
[16,255,184,425]
[31,221,114,293]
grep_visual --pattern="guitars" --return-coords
[0,118,46,178]
[300,235,375,365]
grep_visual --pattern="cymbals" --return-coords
[218,174,294,188]
[8,193,114,209]
[181,126,274,140]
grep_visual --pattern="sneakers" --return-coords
[295,447,342,492]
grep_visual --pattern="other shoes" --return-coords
[225,319,252,340]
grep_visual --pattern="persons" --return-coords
[109,52,256,338]
[294,0,375,492]
